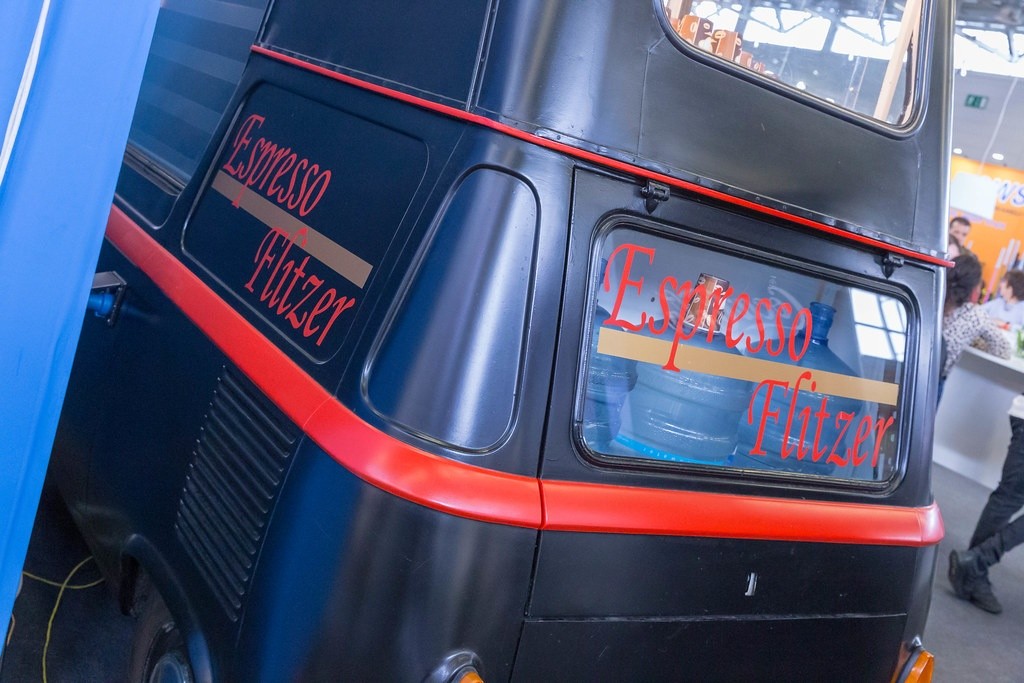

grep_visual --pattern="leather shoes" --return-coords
[947,547,1002,614]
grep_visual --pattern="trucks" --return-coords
[3,0,956,682]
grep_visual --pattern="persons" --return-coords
[934,254,1012,414]
[980,271,1024,333]
[947,390,1024,615]
[949,216,978,261]
[948,234,961,261]
